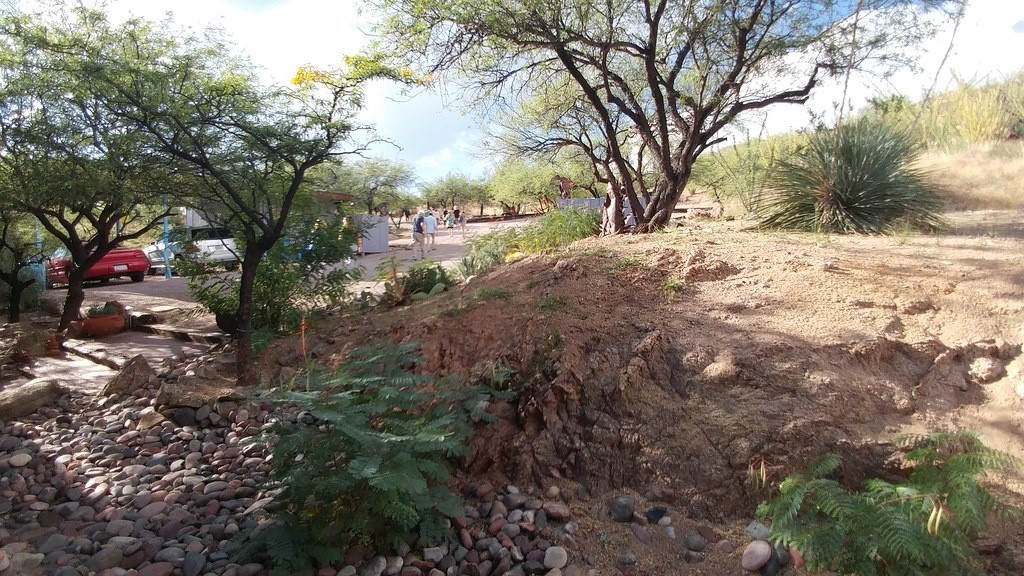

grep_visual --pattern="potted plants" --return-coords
[172,200,380,337]
[85,304,125,338]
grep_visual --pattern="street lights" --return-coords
[141,143,172,280]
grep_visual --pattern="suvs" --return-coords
[142,225,239,273]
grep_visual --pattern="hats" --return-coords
[447,210,451,214]
[459,213,465,216]
[425,210,430,215]
[418,210,425,214]
[560,202,565,206]
[454,206,459,210]
[452,210,454,213]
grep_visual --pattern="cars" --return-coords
[44,239,148,290]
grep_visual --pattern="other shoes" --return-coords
[432,248,436,251]
[427,250,430,252]
[413,258,417,261]
[421,257,425,259]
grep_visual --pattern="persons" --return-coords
[412,210,439,261]
[443,206,467,238]
[404,210,411,221]
